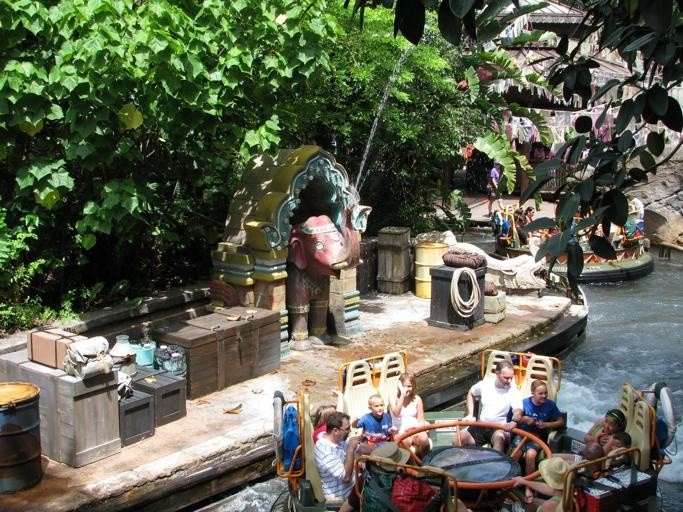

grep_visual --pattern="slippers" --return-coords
[524,494,533,504]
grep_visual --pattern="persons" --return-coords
[314,411,366,500]
[499,457,572,511]
[388,370,431,467]
[311,403,336,444]
[453,359,525,454]
[502,380,564,505]
[553,443,606,480]
[552,408,625,462]
[601,432,631,457]
[500,198,642,251]
[487,158,503,218]
[336,440,472,512]
[352,394,396,448]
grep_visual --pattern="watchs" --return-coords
[510,418,519,424]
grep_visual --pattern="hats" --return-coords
[540,458,574,490]
[366,442,410,474]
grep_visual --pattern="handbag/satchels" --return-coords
[63,336,114,380]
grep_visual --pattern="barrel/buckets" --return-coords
[0,381,42,497]
[109,335,138,378]
[155,345,188,400]
[414,240,449,299]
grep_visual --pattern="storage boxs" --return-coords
[150,304,280,402]
[0,326,188,469]
